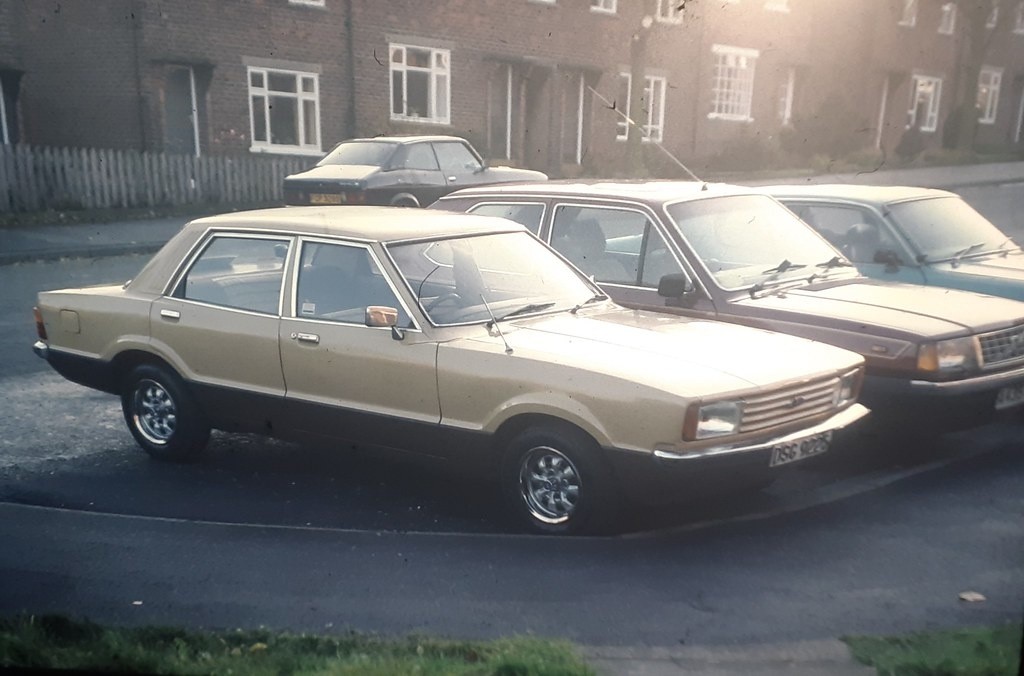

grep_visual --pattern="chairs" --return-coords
[188,243,461,329]
[570,216,633,284]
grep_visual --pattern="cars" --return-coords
[282,137,545,211]
[431,178,1021,456]
[35,205,873,536]
[755,186,1024,307]
